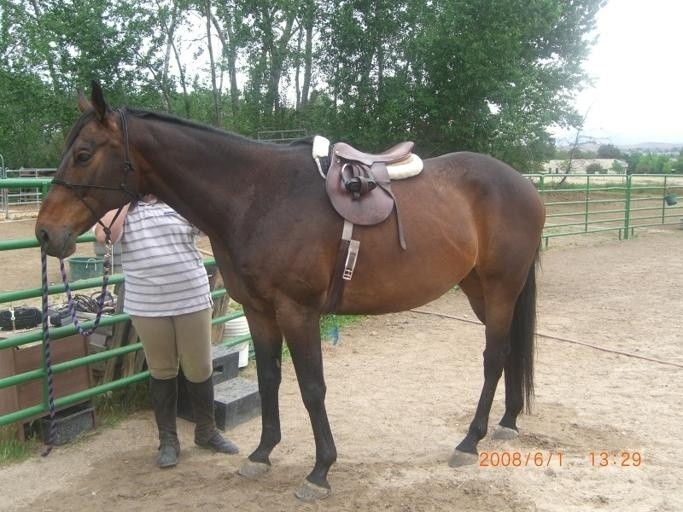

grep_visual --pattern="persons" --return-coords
[92,193,239,468]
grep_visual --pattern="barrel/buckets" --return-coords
[222,317,250,368]
[664,193,676,205]
[65,256,106,282]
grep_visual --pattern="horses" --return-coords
[36,80,546,501]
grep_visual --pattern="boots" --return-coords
[184,373,239,455]
[150,375,180,468]
